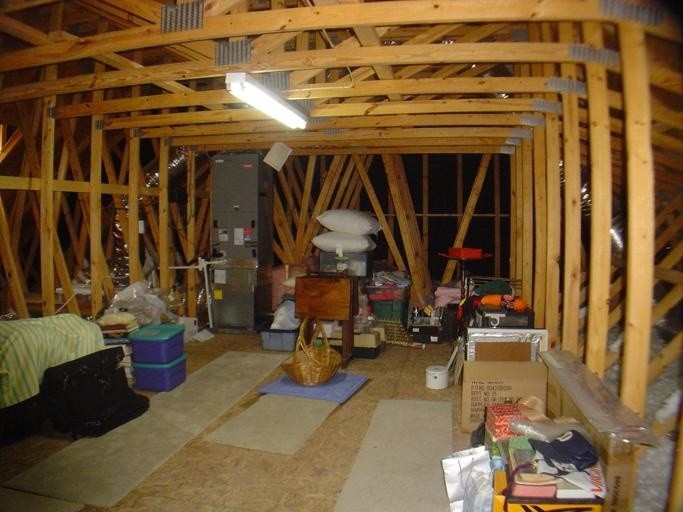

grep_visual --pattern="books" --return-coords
[191,327,215,344]
[99,318,139,386]
[495,432,597,500]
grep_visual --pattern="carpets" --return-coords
[1,349,453,512]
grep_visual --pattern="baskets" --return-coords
[280,313,342,387]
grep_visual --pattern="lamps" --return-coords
[438,248,493,301]
[225,72,308,132]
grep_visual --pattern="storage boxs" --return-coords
[368,299,410,323]
[460,339,548,434]
[318,250,373,277]
[128,323,190,392]
[260,329,299,351]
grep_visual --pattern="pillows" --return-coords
[310,209,381,254]
